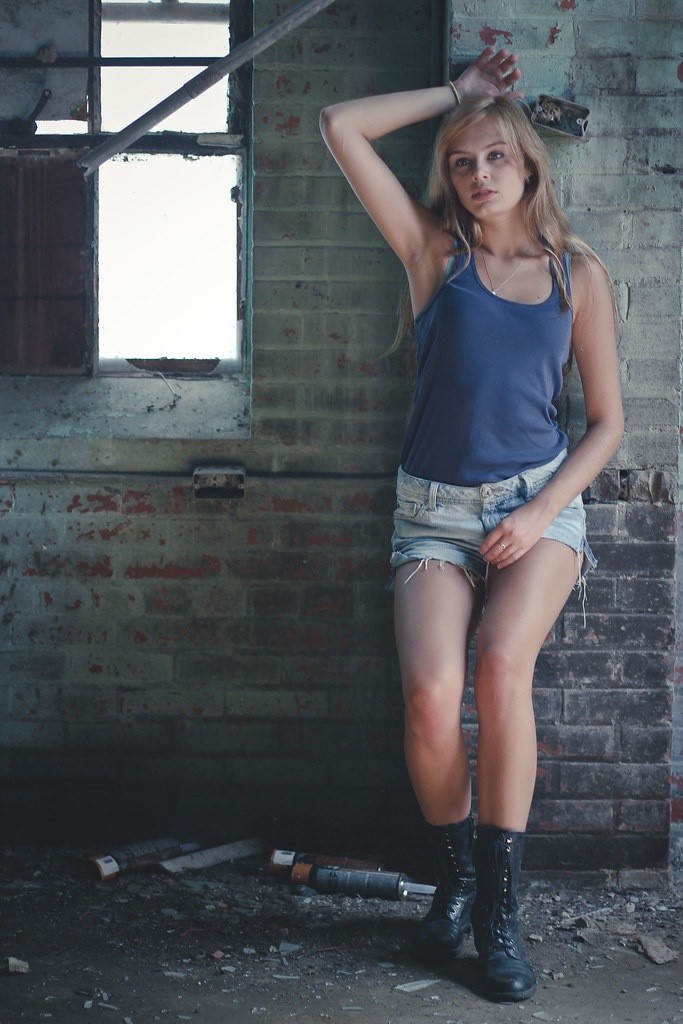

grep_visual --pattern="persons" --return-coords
[319,46,624,1001]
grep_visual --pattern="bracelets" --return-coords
[449,81,461,105]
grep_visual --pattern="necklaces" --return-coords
[475,235,535,295]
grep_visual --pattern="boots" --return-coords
[410,810,479,960]
[474,822,536,1001]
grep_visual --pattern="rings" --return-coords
[498,542,505,549]
